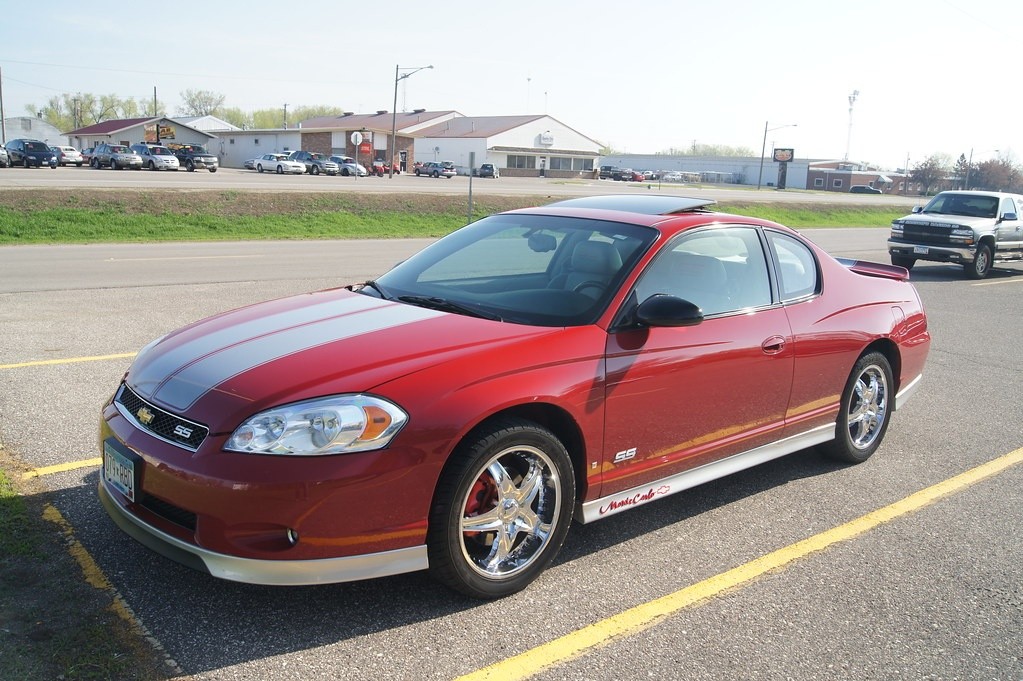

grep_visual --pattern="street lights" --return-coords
[758,121,798,190]
[389,65,433,178]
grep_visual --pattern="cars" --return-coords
[246,150,369,176]
[0,139,83,169]
[479,163,499,179]
[97,193,932,600]
[88,145,219,173]
[415,161,457,178]
[600,165,702,182]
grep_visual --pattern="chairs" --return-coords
[564,240,622,300]
[663,253,737,314]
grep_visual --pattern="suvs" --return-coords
[887,190,1023,280]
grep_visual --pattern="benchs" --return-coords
[667,250,789,304]
